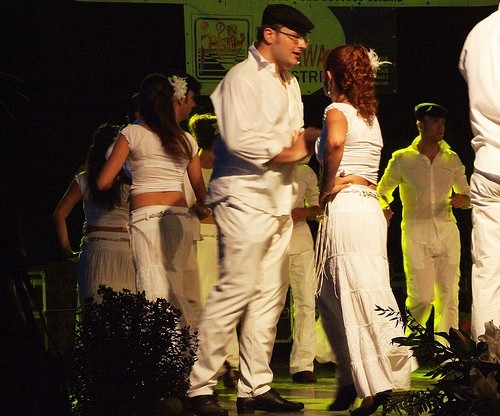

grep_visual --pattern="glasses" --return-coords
[276,30,310,42]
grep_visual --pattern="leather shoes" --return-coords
[236,388,304,414]
[192,395,228,416]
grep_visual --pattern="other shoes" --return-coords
[224,371,238,388]
[292,371,317,383]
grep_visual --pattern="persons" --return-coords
[96,73,208,366]
[458,0,500,346]
[188,113,240,388]
[374,102,472,365]
[289,152,321,384]
[172,74,202,125]
[183,4,321,416]
[53,122,136,351]
[315,43,419,416]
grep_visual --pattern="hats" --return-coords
[262,4,314,33]
[414,103,449,119]
[179,72,203,94]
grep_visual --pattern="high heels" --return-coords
[350,389,392,416]
[328,382,358,411]
